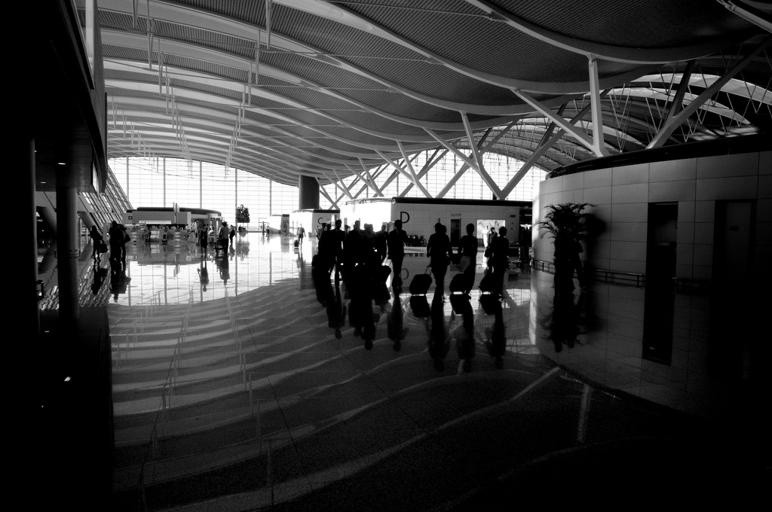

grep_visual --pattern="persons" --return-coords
[121,223,131,262]
[297,223,305,244]
[325,283,507,374]
[216,253,232,288]
[108,220,124,265]
[107,258,131,304]
[87,223,104,264]
[197,255,211,294]
[228,224,236,249]
[90,261,108,297]
[311,219,514,294]
[196,225,208,255]
[219,222,229,256]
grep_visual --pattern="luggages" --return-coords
[409,264,433,295]
[479,293,498,315]
[448,273,468,293]
[373,282,390,305]
[478,271,498,293]
[409,295,431,318]
[377,259,392,282]
[449,293,467,314]
